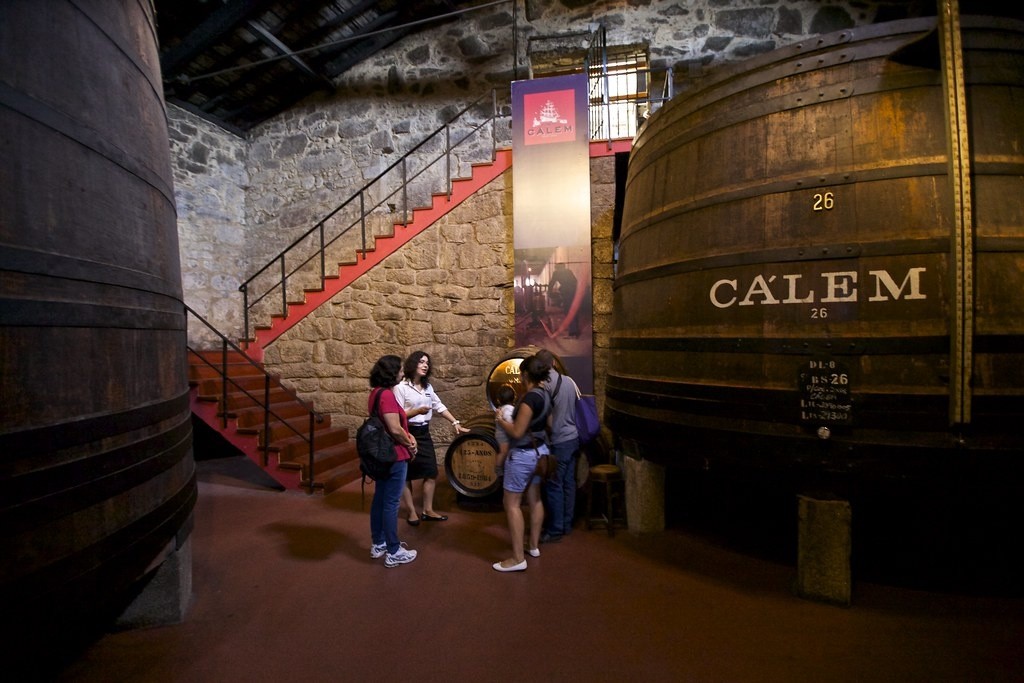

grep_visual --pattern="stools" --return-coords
[585,464,627,537]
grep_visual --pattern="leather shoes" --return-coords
[420,513,448,522]
[492,559,527,572]
[523,548,541,557]
[407,513,420,527]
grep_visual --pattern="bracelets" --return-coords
[451,421,459,425]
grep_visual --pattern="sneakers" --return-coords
[384,542,418,568]
[370,541,388,559]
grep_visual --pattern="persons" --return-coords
[368,355,417,568]
[394,352,471,525]
[493,356,552,571]
[495,386,515,479]
[536,349,582,542]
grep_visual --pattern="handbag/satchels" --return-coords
[564,376,600,442]
[529,432,558,480]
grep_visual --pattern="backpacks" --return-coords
[356,387,407,491]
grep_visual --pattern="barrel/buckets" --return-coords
[443,415,499,497]
